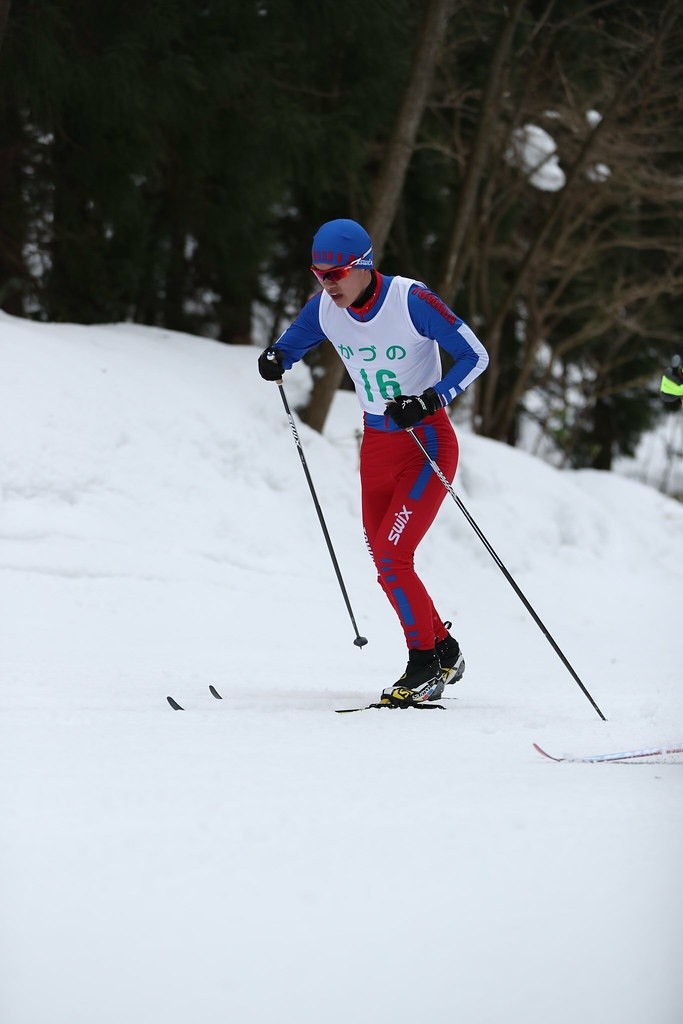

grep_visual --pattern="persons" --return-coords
[258,218,491,702]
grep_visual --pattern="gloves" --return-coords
[258,347,285,382]
[384,386,444,429]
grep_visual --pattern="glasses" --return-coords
[310,264,352,283]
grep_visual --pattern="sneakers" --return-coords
[380,637,445,704]
[434,620,466,686]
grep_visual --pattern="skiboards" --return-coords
[161,679,462,717]
[530,740,682,766]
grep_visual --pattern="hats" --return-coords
[311,219,373,270]
